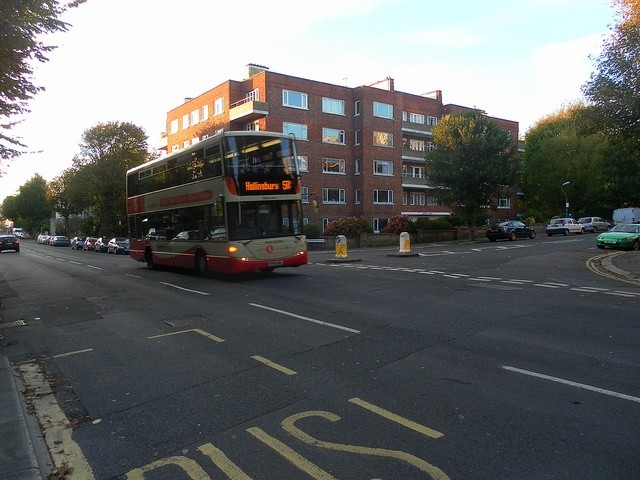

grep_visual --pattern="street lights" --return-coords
[561,182,570,218]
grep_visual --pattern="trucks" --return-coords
[13,228,22,236]
[613,208,640,227]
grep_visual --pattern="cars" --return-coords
[0,235,19,253]
[596,223,640,251]
[94,236,113,253]
[53,236,69,247]
[577,217,612,233]
[546,217,585,236]
[71,236,86,250]
[108,237,130,255]
[83,237,99,251]
[37,235,55,246]
[15,231,32,240]
[486,220,536,242]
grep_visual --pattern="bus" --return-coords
[126,131,307,278]
